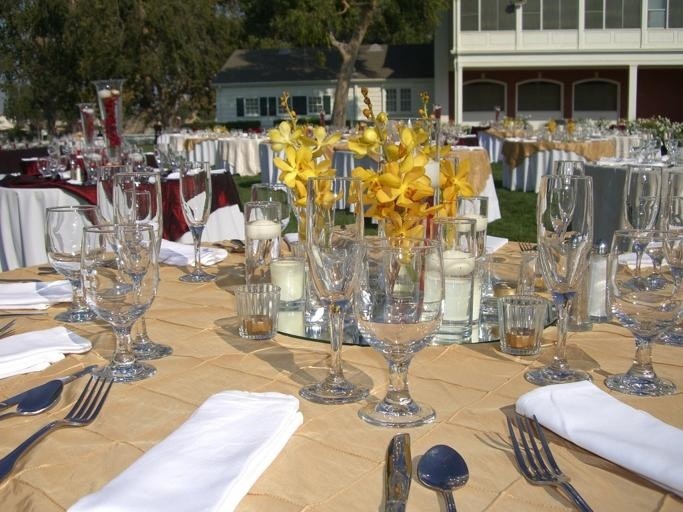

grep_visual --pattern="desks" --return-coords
[0,234,683,512]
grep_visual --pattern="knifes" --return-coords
[382,433,412,512]
[0,364,99,411]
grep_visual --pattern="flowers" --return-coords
[251,84,490,334]
[499,120,596,138]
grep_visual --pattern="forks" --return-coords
[0,376,114,482]
[507,414,594,512]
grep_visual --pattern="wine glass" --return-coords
[350,234,444,429]
[177,162,218,283]
[621,163,662,269]
[44,204,103,323]
[111,172,174,363]
[602,228,682,397]
[523,173,594,387]
[79,221,161,383]
[298,176,370,406]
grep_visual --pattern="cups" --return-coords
[234,283,282,340]
[267,256,307,312]
[427,216,478,346]
[495,296,548,356]
[243,201,283,293]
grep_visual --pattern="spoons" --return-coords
[417,444,470,512]
[0,379,63,420]
